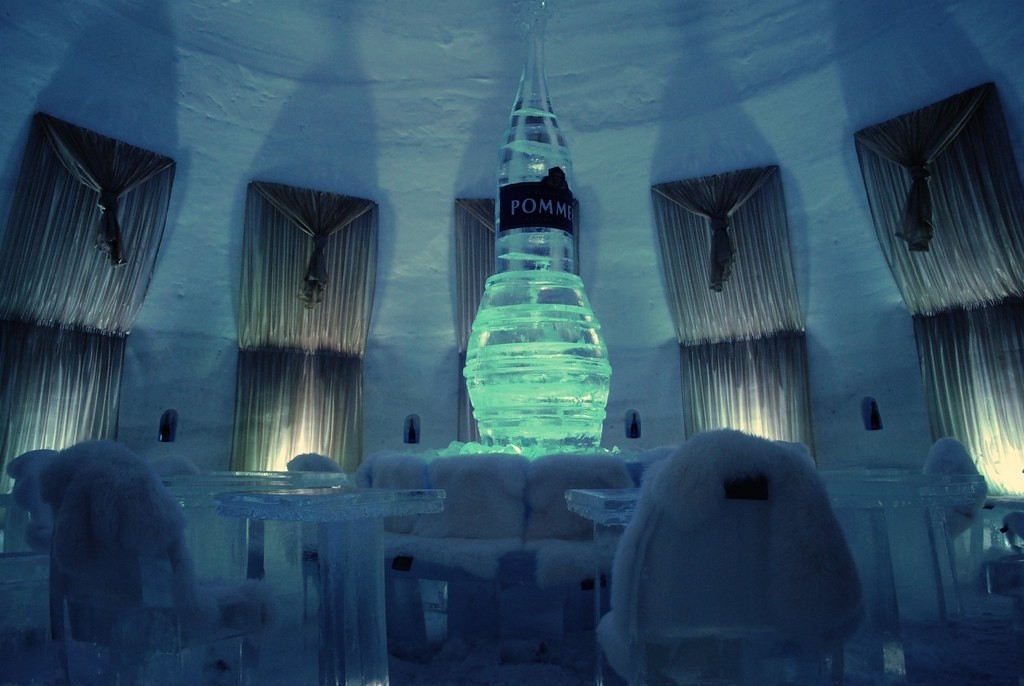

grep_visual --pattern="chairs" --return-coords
[0,428,1024,686]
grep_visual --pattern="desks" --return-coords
[565,487,638,686]
[160,465,350,577]
[814,463,987,686]
[215,484,447,686]
